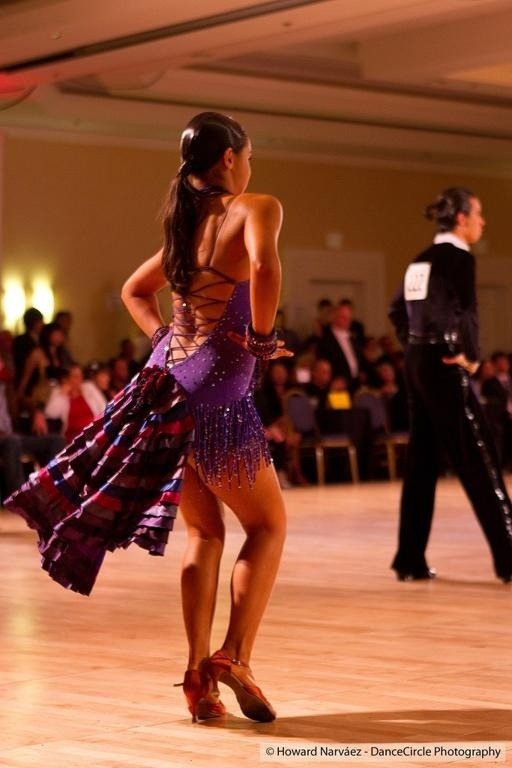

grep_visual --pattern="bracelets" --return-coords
[243,323,279,359]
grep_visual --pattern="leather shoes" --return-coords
[392,552,437,582]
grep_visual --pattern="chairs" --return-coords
[353,386,411,481]
[282,388,358,484]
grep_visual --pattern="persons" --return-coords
[386,189,511,587]
[2,294,512,501]
[0,111,293,723]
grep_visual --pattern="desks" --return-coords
[319,407,369,460]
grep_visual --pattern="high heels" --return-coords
[183,650,277,723]
